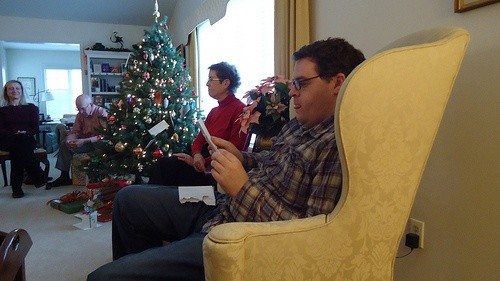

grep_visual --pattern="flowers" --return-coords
[233,75,292,140]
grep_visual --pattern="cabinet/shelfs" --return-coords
[83,50,134,113]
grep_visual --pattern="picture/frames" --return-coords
[17,77,36,96]
[39,113,45,122]
[454,0,500,14]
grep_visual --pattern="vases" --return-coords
[262,118,286,138]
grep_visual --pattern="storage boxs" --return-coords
[88,184,120,200]
[50,199,90,215]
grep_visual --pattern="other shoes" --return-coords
[47,177,72,187]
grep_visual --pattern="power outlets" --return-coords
[410,217,424,249]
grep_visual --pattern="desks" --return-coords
[39,120,74,128]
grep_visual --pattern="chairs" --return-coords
[0,130,52,187]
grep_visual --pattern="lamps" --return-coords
[32,87,55,115]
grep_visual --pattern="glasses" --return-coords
[209,77,222,82]
[291,74,325,90]
[77,105,91,111]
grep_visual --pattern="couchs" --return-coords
[202,25,472,281]
[56,123,90,186]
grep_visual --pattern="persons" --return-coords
[149,61,250,187]
[88,37,367,281]
[50,94,109,187]
[0,80,48,198]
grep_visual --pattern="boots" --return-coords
[12,175,24,198]
[29,166,53,187]
